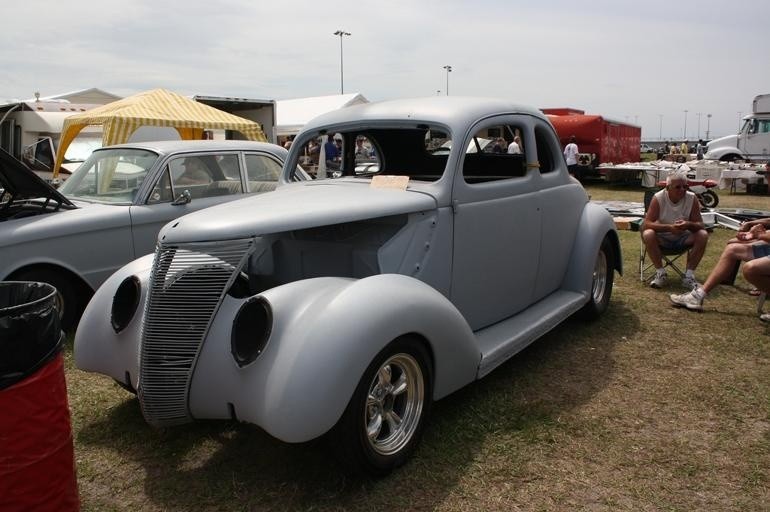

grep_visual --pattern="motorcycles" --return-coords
[655,167,719,208]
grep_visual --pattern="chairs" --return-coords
[638,185,694,282]
[394,152,524,184]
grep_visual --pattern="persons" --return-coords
[668,217,770,323]
[638,172,710,293]
[659,135,706,162]
[284,140,292,151]
[491,137,503,152]
[305,135,378,164]
[507,136,522,153]
[562,135,581,176]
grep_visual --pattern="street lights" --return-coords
[443,66,452,96]
[334,31,351,95]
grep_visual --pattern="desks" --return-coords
[722,169,765,196]
[595,165,673,190]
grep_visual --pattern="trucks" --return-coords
[702,94,770,162]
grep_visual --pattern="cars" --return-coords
[0,140,313,342]
[72,96,622,469]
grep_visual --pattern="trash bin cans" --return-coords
[0,278,82,511]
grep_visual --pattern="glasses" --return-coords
[672,183,689,189]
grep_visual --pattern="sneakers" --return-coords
[761,314,770,322]
[682,277,697,289]
[651,272,667,288]
[670,284,704,311]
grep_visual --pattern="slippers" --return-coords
[750,287,760,295]
